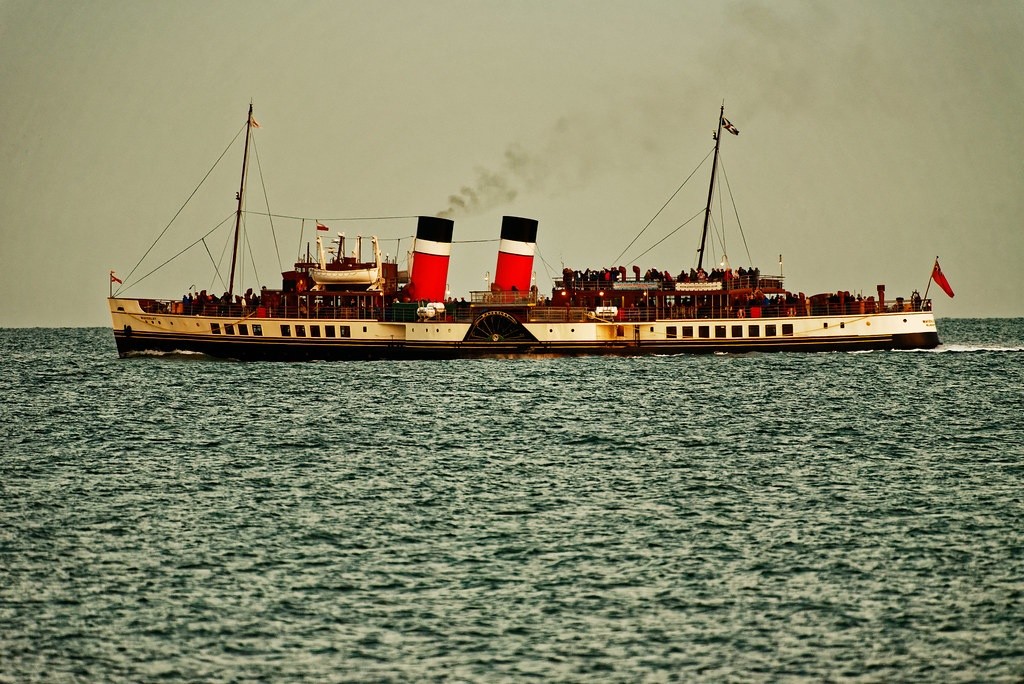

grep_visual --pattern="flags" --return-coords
[932,258,954,298]
[316,219,329,231]
[111,270,115,273]
[250,113,262,129]
[722,115,740,136]
[111,275,122,284]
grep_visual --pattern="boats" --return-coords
[310,236,383,285]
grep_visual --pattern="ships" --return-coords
[106,97,944,362]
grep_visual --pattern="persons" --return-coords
[184,267,877,322]
